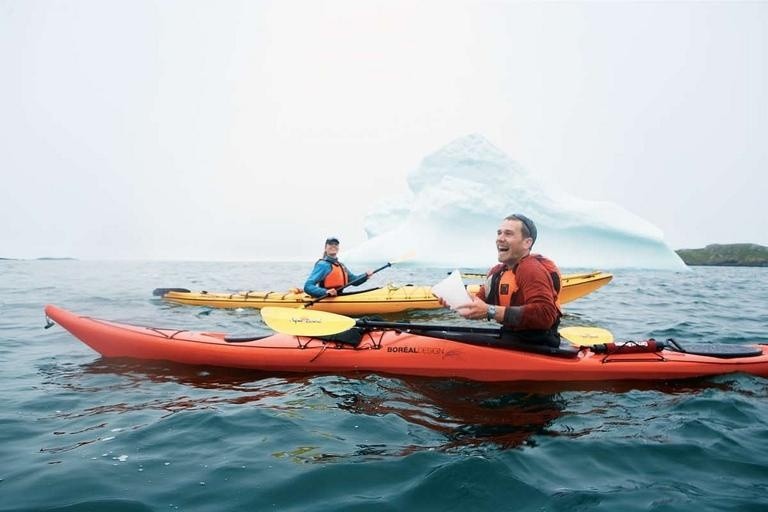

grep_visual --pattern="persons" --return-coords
[438,213,563,347]
[304,237,374,298]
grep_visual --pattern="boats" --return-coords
[153,269,613,319]
[42,304,767,381]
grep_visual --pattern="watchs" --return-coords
[487,304,496,321]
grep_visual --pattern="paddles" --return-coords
[260,307,614,348]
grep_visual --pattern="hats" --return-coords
[326,235,339,245]
[513,213,537,241]
